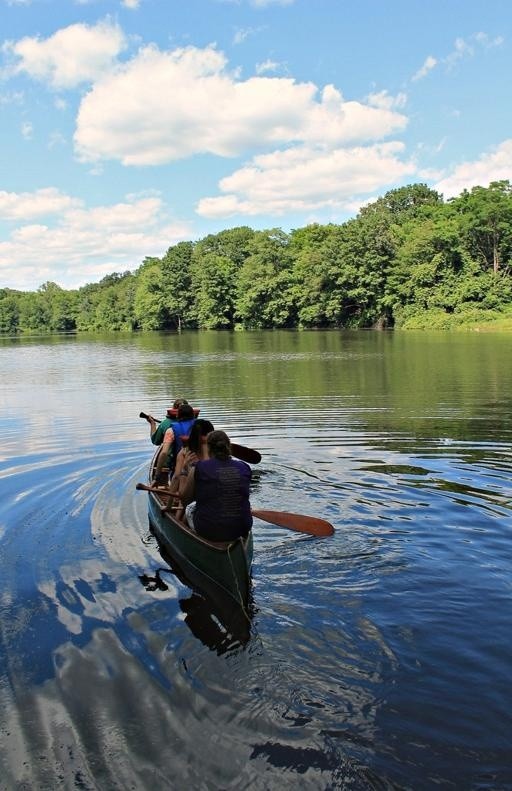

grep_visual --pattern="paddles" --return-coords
[136,483,335,536]
[139,412,261,464]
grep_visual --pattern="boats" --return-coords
[147,445,254,612]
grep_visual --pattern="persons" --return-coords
[179,430,253,541]
[148,399,193,445]
[169,419,232,521]
[151,405,198,481]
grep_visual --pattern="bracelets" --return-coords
[180,469,188,476]
